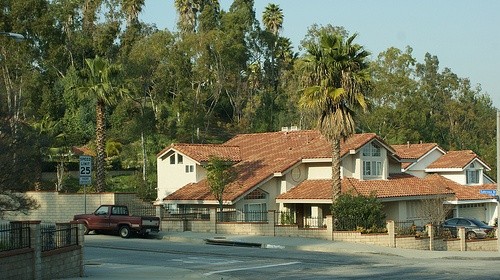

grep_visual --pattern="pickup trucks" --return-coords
[73,204,160,238]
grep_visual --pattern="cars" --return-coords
[441,217,496,240]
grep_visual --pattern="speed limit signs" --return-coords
[79,155,92,185]
